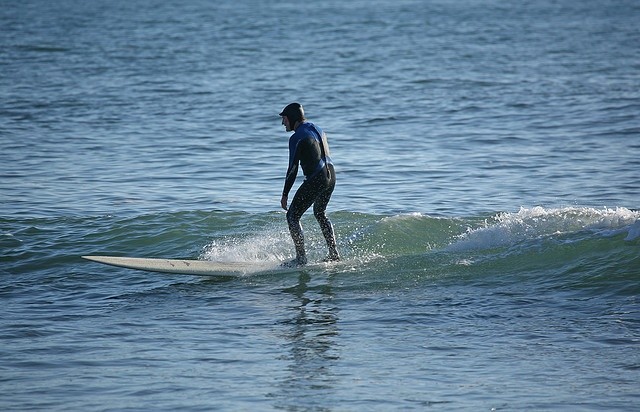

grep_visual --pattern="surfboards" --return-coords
[80,249,387,279]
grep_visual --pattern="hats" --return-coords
[279,103,305,130]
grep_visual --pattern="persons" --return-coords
[279,101,340,267]
